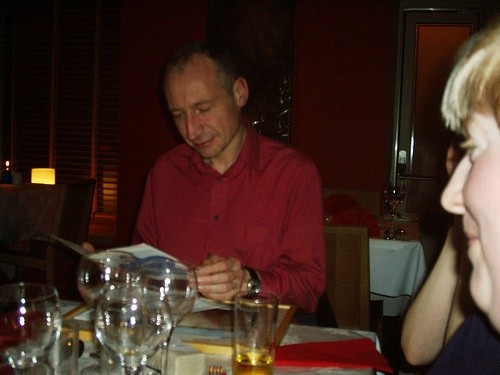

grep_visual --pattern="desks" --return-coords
[58,300,385,375]
[320,210,440,316]
[1,183,60,281]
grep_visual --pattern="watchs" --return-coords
[241,265,261,299]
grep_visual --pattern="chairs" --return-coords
[0,178,96,285]
[323,227,384,340]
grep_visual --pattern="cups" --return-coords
[77,249,140,375]
[0,282,63,375]
[232,290,277,366]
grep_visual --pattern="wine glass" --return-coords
[137,257,194,375]
[380,184,406,222]
[384,221,407,241]
[94,285,173,375]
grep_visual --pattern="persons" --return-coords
[400,15,500,375]
[81,41,327,313]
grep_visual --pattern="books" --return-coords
[40,230,195,284]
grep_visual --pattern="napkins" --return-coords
[275,338,391,373]
[330,194,380,236]
